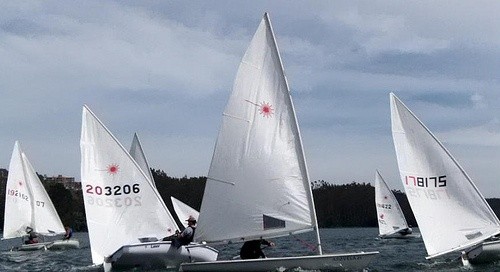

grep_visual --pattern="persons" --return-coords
[25,229,38,245]
[403,225,413,234]
[63,226,73,240]
[240,239,274,260]
[163,217,197,245]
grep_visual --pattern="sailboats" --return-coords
[373,168,412,238]
[78,11,380,272]
[0,139,81,251]
[389,91,500,269]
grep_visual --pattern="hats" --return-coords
[185,215,196,221]
